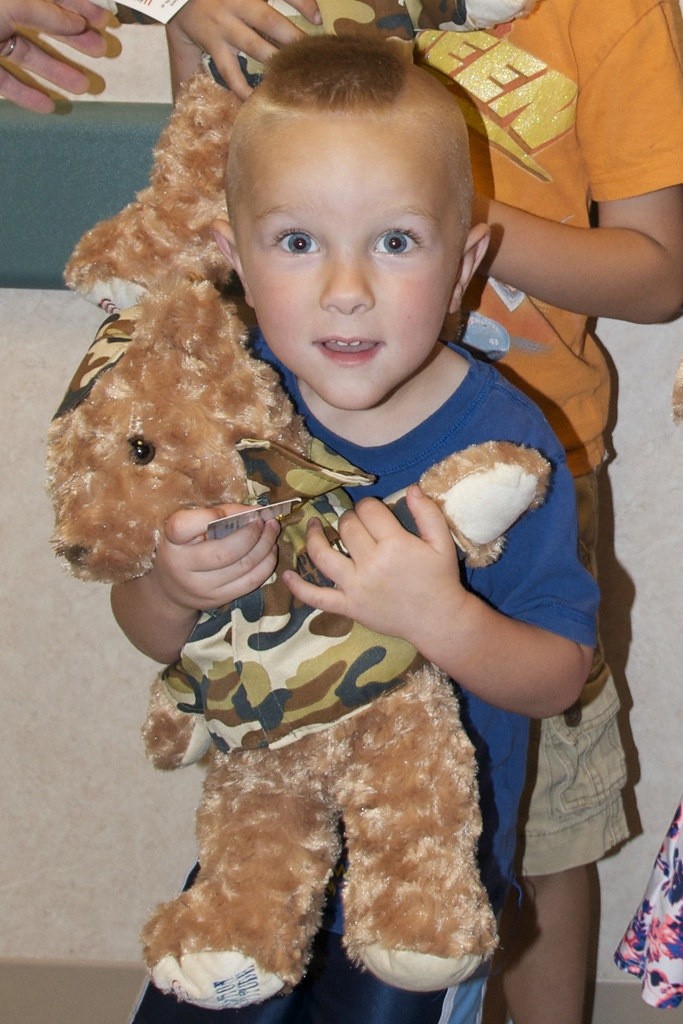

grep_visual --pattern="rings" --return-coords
[3,36,16,57]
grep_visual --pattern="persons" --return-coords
[164,0,683,1024]
[0,0,121,114]
[119,33,601,1024]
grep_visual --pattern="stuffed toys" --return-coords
[50,294,553,1010]
[64,0,534,311]
